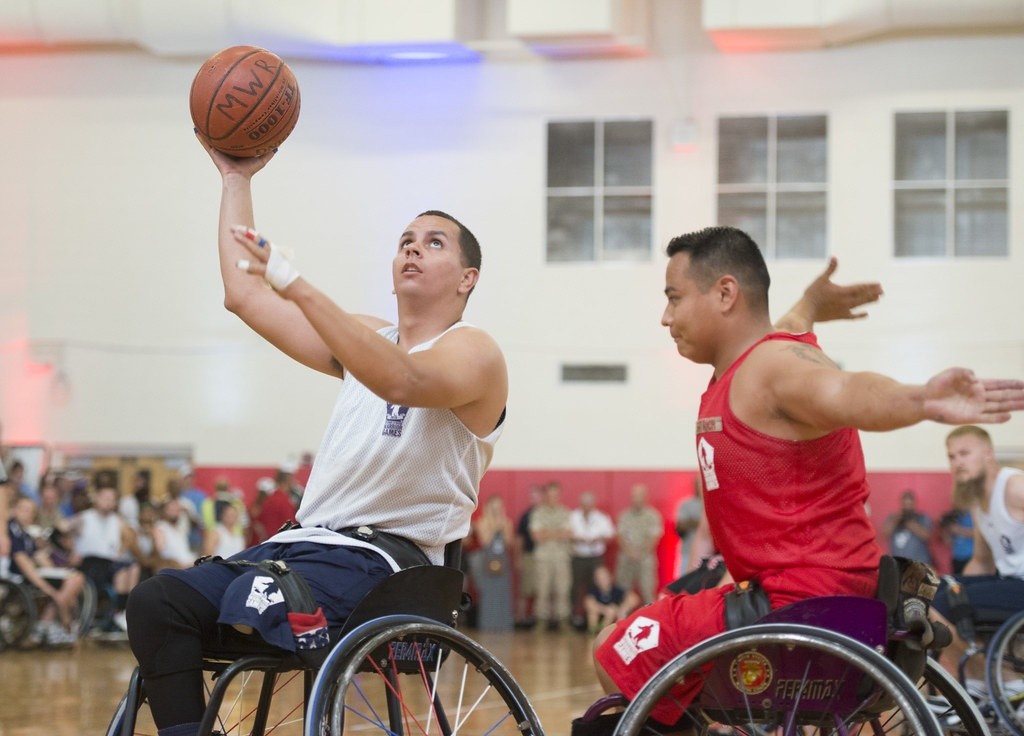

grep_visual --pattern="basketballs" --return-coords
[188,44,303,161]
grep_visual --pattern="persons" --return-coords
[592,226,1024,736]
[883,423,1024,715]
[127,128,508,736]
[0,449,312,652]
[468,477,727,635]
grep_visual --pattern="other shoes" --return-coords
[9,622,129,652]
[520,611,608,632]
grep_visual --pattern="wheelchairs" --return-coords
[928,575,1024,736]
[104,520,544,736]
[0,567,97,652]
[571,555,991,736]
